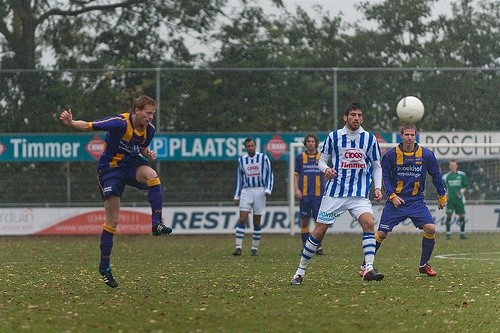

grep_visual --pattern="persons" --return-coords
[441,160,469,240]
[60,96,173,288]
[233,138,274,256]
[358,124,446,279]
[294,135,325,255]
[290,102,384,285]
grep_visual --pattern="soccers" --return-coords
[396,96,425,123]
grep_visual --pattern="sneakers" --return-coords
[300,249,304,255]
[447,233,469,239]
[363,269,384,282]
[291,269,305,285]
[99,268,118,288]
[152,225,172,237]
[316,247,323,255]
[233,249,242,256]
[359,265,366,277]
[418,264,436,277]
[250,249,257,256]
